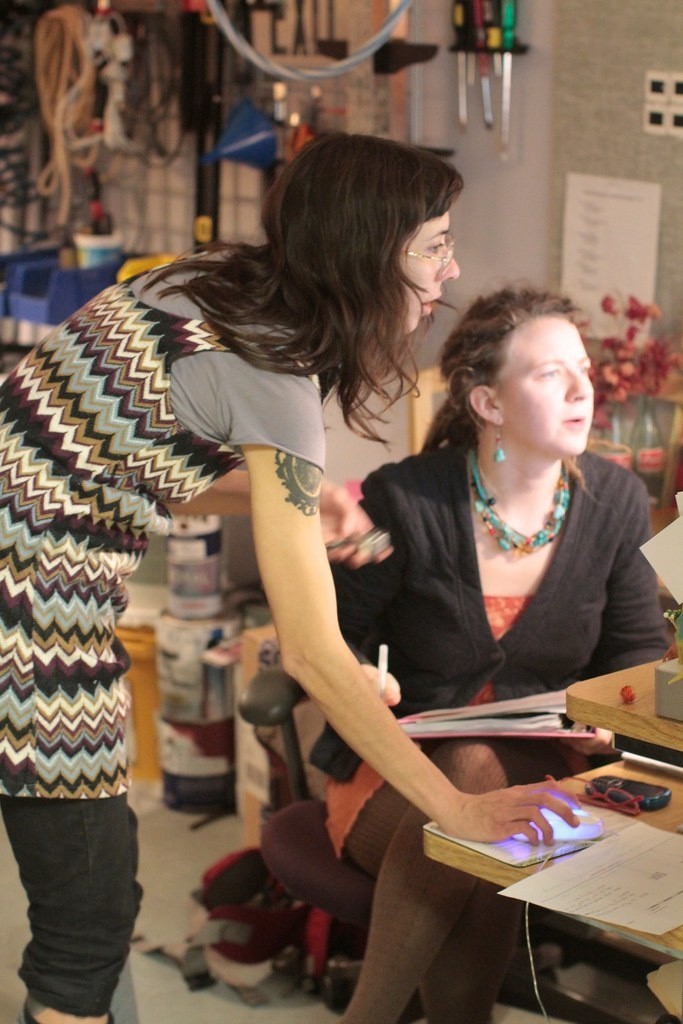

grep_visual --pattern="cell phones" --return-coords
[585,775,672,811]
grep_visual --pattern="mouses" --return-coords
[513,807,604,844]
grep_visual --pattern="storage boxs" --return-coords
[0,250,175,324]
[235,630,328,846]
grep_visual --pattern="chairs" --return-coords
[236,667,550,1024]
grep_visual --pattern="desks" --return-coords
[423,660,683,1024]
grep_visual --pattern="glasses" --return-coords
[399,234,454,271]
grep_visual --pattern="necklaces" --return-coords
[469,449,569,558]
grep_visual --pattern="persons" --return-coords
[1,134,579,1022]
[308,292,668,1023]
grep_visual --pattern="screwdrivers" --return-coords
[452,0,519,165]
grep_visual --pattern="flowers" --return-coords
[584,297,683,432]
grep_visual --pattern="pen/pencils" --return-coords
[379,644,389,694]
